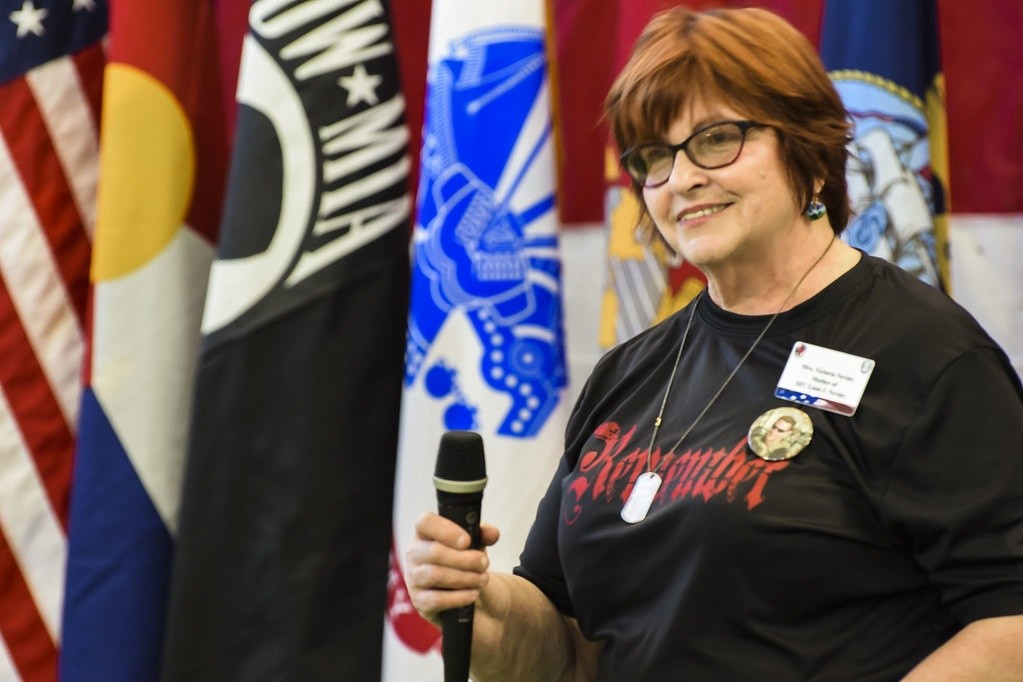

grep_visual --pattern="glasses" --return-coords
[623,120,770,188]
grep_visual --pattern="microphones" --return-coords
[431,430,488,682]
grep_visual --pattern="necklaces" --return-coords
[620,226,837,525]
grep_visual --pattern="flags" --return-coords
[3,1,957,680]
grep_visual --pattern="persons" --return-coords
[753,417,797,460]
[406,9,1021,681]
